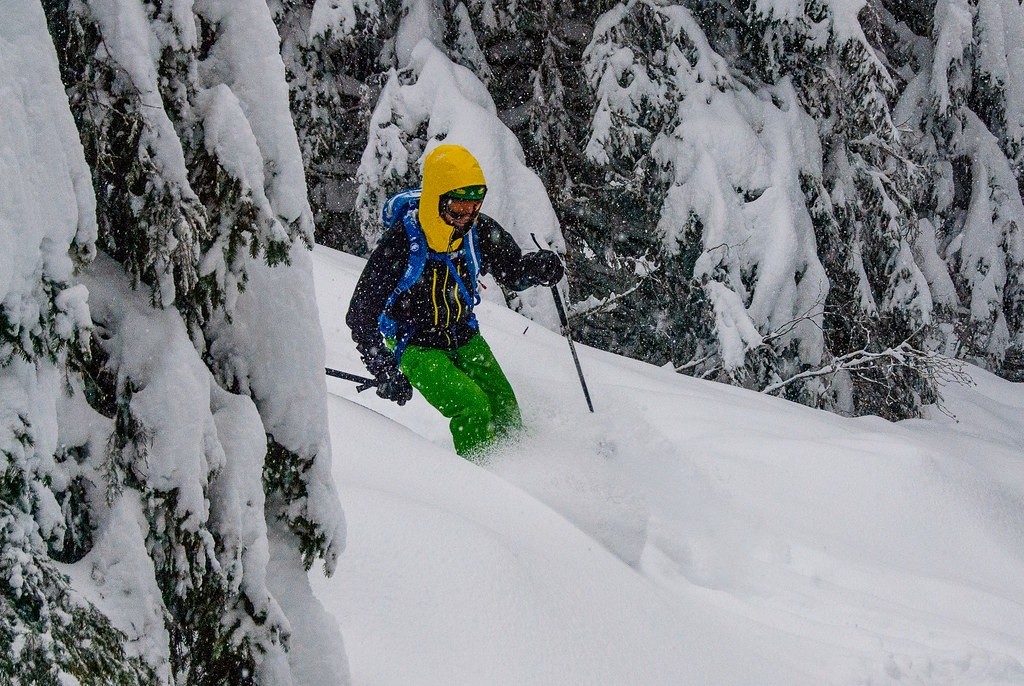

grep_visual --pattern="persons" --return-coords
[346,145,564,458]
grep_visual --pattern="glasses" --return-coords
[442,197,483,219]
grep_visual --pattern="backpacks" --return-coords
[377,188,481,339]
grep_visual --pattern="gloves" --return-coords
[375,368,413,406]
[530,249,564,284]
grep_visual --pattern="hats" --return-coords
[445,185,486,200]
